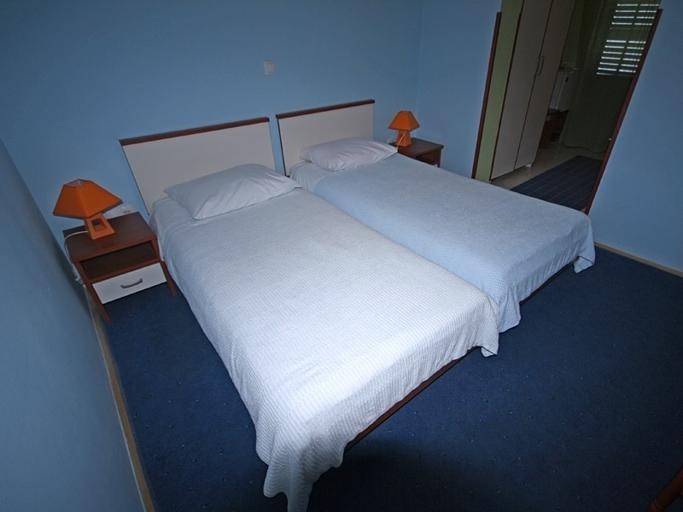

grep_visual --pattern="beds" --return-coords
[120,117,500,510]
[275,100,596,333]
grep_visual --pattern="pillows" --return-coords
[302,138,397,171]
[163,163,302,222]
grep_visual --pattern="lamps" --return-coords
[387,111,421,147]
[52,176,123,240]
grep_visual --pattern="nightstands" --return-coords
[388,136,443,168]
[63,212,179,322]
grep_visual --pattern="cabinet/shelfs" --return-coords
[474,0,574,180]
